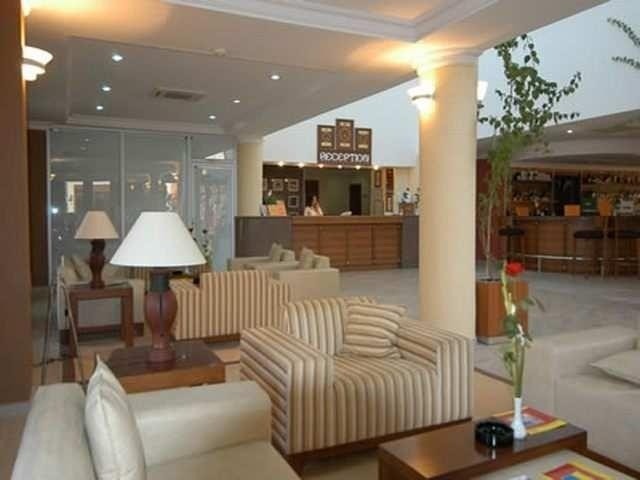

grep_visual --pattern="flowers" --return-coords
[397,183,422,209]
[495,255,549,400]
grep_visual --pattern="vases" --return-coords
[509,395,528,441]
[398,201,416,217]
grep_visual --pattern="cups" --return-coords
[192,257,209,284]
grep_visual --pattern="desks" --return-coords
[374,401,640,480]
[83,335,230,401]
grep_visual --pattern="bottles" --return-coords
[587,170,639,185]
[512,168,551,216]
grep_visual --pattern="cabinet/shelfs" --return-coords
[505,162,640,223]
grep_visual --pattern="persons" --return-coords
[303,194,323,217]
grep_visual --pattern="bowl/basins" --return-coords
[474,421,513,447]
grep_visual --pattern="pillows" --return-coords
[341,300,412,362]
[78,349,148,480]
[588,342,639,389]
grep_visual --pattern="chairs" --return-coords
[510,321,640,475]
[8,373,304,480]
[238,293,476,478]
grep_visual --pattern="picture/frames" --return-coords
[374,169,382,188]
[262,177,300,209]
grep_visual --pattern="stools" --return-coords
[568,227,607,278]
[607,225,640,281]
[497,226,527,272]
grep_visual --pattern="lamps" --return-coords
[103,208,208,371]
[474,78,489,124]
[405,79,436,119]
[72,208,124,291]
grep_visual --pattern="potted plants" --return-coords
[470,28,588,346]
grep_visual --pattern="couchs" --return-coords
[54,237,345,352]
